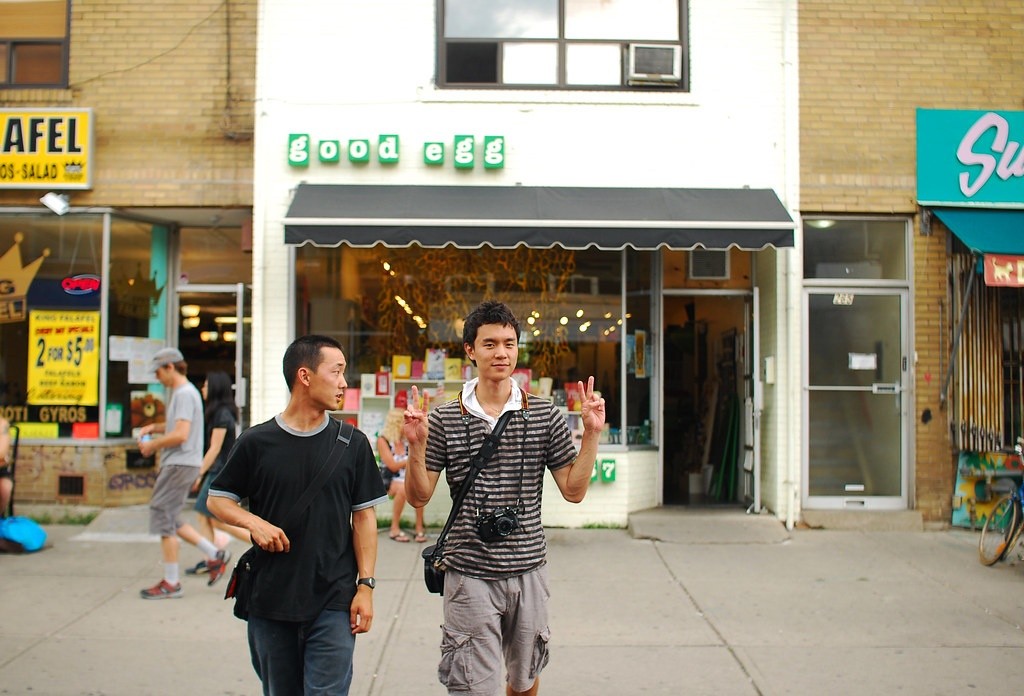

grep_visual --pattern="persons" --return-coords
[137,348,231,600]
[377,408,428,543]
[0,416,13,551]
[206,333,388,696]
[402,298,605,696]
[185,369,252,575]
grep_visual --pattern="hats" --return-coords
[148,347,184,375]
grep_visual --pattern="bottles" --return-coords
[141,432,153,455]
[376,366,389,395]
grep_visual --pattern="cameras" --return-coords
[475,507,521,544]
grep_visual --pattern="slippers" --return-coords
[414,528,427,543]
[389,530,410,542]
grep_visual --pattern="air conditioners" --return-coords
[622,43,684,86]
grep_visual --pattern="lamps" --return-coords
[39,192,72,216]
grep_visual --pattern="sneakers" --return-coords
[139,578,184,599]
[205,549,231,586]
[184,561,212,576]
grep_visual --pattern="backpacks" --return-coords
[0,516,48,551]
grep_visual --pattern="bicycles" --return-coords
[978,442,1024,566]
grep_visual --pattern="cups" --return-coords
[537,377,553,397]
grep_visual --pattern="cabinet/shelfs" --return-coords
[321,382,586,454]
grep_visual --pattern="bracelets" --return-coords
[199,473,203,478]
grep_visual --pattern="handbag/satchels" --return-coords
[224,545,257,621]
[421,542,444,597]
[377,467,400,492]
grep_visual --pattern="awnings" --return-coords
[926,206,1024,410]
[278,181,796,252]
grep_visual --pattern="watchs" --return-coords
[358,577,375,590]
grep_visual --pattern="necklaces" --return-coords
[476,393,504,415]
